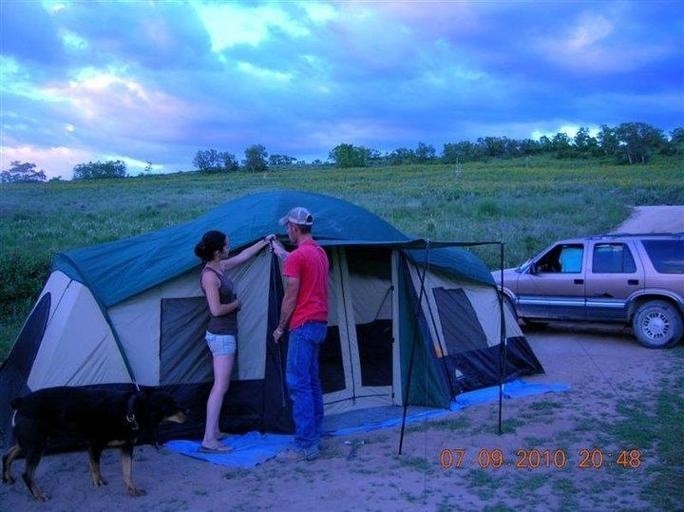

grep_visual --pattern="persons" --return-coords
[192,230,275,453]
[268,206,330,464]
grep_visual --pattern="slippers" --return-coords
[199,432,234,452]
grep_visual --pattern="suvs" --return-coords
[491,232,683,349]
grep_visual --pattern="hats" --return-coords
[278,207,314,225]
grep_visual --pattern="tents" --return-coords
[2,187,547,461]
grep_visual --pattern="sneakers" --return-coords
[275,446,321,463]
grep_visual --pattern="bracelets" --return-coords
[275,328,284,335]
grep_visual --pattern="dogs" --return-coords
[0,386,188,503]
